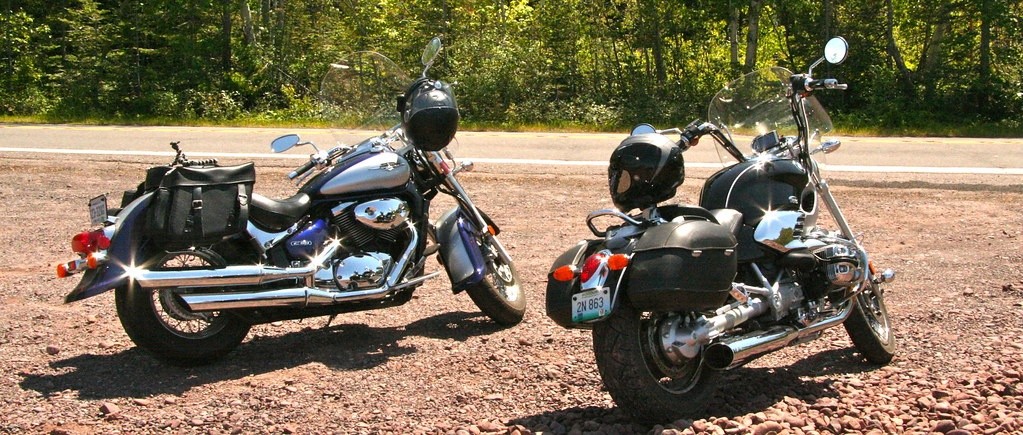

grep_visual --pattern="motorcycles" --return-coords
[546,35,898,424]
[57,35,527,367]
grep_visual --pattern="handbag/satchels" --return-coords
[145,161,257,244]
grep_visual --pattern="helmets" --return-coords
[609,133,685,214]
[401,76,460,150]
